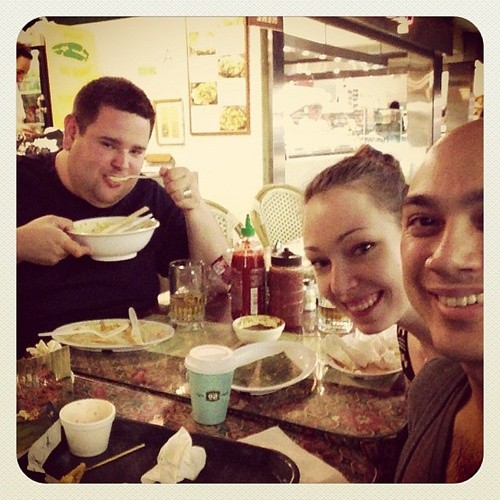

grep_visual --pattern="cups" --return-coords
[317,293,354,336]
[59,398,116,458]
[184,344,237,426]
[170,258,206,331]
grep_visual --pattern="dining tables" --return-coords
[56,274,436,445]
[17,361,378,483]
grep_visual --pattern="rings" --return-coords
[182,189,193,199]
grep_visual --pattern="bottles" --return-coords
[230,215,267,320]
[302,278,317,311]
[267,248,304,333]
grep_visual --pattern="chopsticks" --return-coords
[98,205,153,234]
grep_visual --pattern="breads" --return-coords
[321,332,402,369]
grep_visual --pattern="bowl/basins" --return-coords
[325,356,404,381]
[67,216,160,262]
[228,340,318,396]
[233,315,285,343]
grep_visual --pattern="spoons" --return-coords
[107,175,162,181]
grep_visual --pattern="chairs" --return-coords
[250,182,309,250]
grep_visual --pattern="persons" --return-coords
[376,101,405,131]
[298,104,329,136]
[301,145,445,398]
[16,41,39,149]
[395,117,483,484]
[16,78,232,361]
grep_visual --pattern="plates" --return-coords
[51,318,175,354]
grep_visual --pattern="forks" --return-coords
[37,322,130,339]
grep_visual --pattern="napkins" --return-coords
[139,426,207,484]
[236,425,349,484]
[323,327,396,370]
